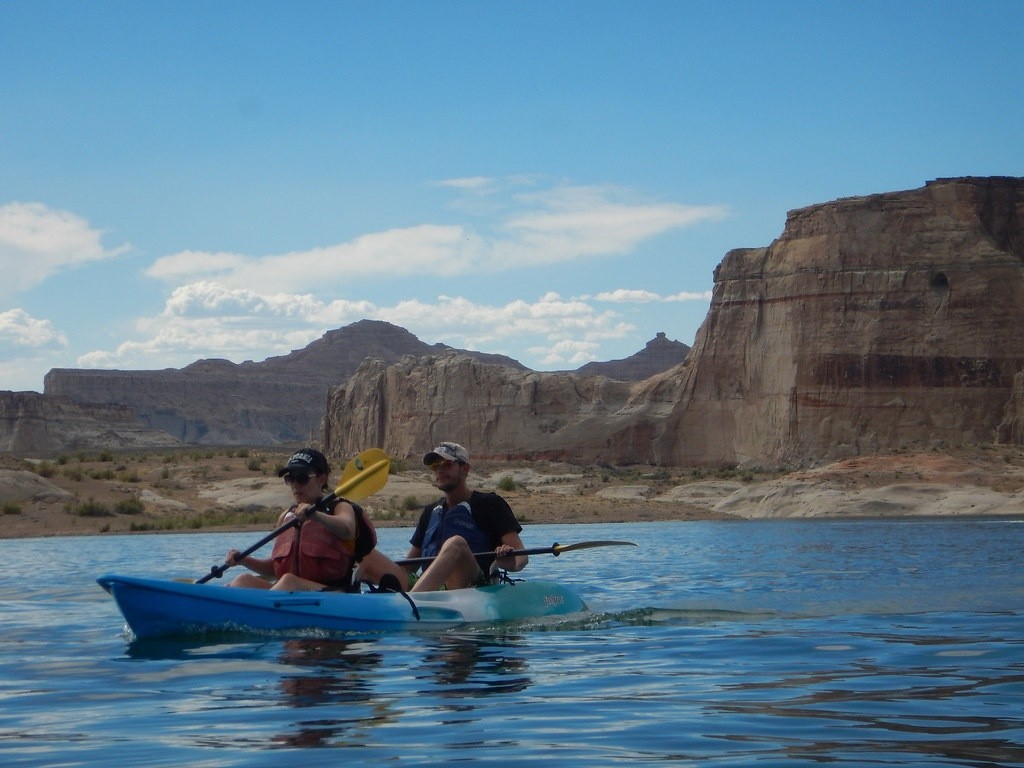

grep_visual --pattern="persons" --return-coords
[225,448,376,591]
[351,441,529,593]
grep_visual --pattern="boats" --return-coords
[95,572,591,641]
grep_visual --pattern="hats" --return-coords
[278,448,327,476]
[422,441,470,465]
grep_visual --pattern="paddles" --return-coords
[389,540,638,564]
[168,447,389,586]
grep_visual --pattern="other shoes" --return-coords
[378,573,403,593]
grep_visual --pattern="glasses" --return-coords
[430,459,458,472]
[284,472,317,486]
